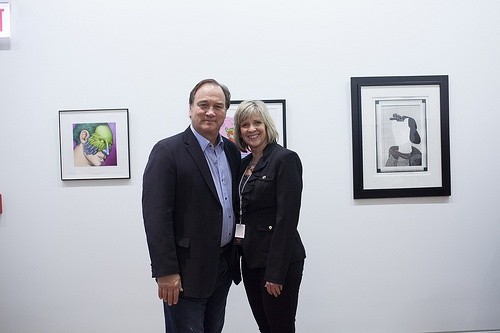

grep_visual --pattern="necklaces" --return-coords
[248,158,257,172]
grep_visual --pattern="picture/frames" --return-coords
[58,109,131,181]
[218,99,286,148]
[351,74,452,200]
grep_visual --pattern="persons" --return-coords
[234,100,306,333]
[142,78,241,333]
